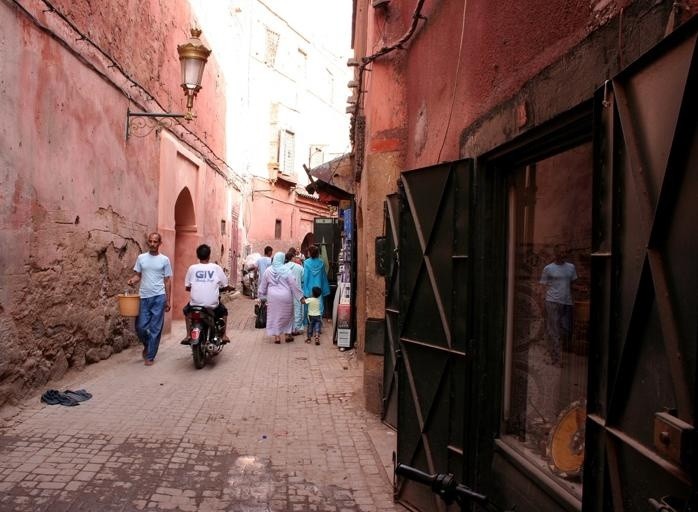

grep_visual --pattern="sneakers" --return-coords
[274,330,320,345]
[142,350,148,357]
[145,359,152,365]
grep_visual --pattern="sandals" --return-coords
[222,336,230,342]
[180,337,192,344]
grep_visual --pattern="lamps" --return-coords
[126,29,212,139]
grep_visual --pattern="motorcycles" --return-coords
[179,283,234,369]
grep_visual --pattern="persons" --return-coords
[302,287,323,346]
[257,251,307,344]
[536,244,591,369]
[257,245,332,336]
[181,243,235,345]
[127,232,171,366]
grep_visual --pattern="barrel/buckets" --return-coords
[117,283,140,317]
[573,289,590,322]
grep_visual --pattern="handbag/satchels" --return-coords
[254,301,267,328]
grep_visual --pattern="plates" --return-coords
[547,399,584,478]
